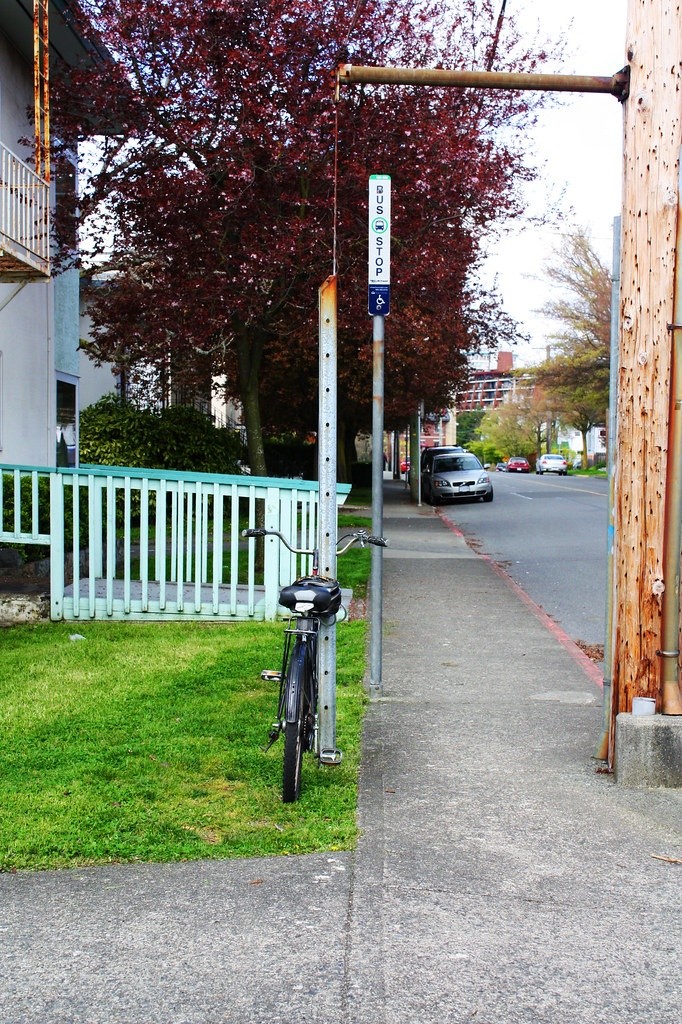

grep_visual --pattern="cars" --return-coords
[496,462,508,472]
[536,453,568,476]
[507,457,531,472]
[423,453,494,504]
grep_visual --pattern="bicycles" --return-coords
[242,528,389,803]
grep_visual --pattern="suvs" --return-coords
[420,446,466,471]
[399,456,411,474]
